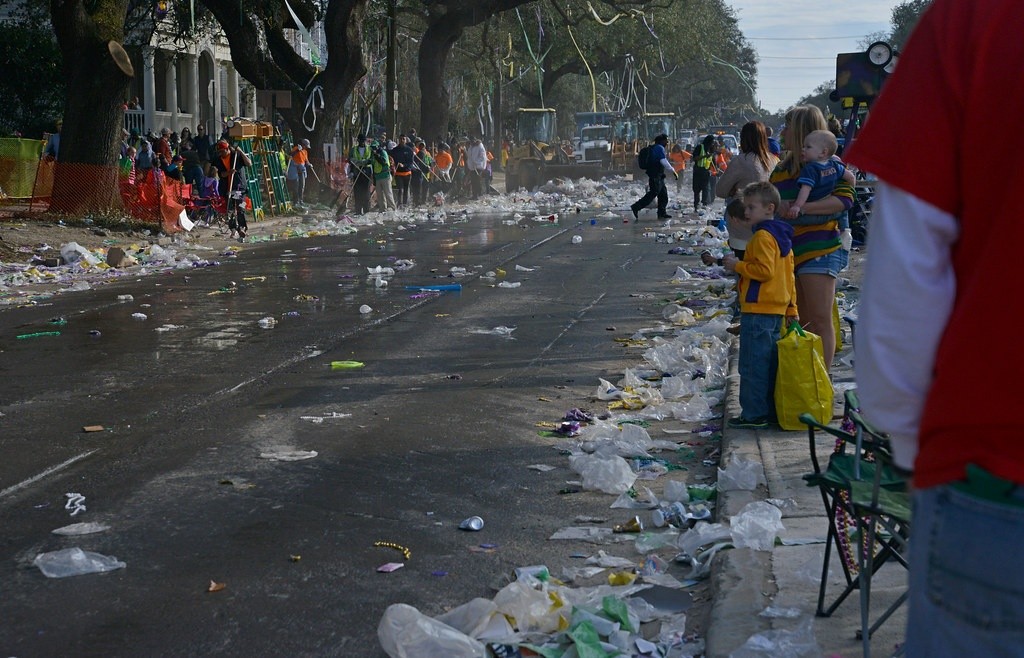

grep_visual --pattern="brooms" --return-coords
[297,144,334,193]
[413,152,468,203]
[335,167,364,218]
[351,161,398,208]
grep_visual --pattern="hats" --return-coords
[217,142,227,151]
[161,128,173,134]
[121,128,130,135]
[359,128,426,146]
[172,155,186,161]
[301,139,311,148]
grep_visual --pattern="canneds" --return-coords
[458,516,484,530]
[613,516,643,532]
[511,564,548,582]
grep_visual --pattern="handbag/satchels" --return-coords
[831,298,842,353]
[773,317,835,431]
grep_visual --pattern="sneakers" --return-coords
[727,414,778,428]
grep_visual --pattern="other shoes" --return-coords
[630,205,638,218]
[657,213,672,219]
[230,227,245,239]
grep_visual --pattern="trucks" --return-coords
[841,97,868,134]
[571,111,741,170]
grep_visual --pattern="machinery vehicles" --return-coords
[505,107,578,193]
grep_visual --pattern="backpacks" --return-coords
[638,144,656,170]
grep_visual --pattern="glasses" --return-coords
[183,131,189,132]
[198,129,204,131]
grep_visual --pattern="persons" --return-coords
[631,134,678,219]
[288,139,314,206]
[840,0,1023,658]
[43,96,252,239]
[348,127,514,214]
[671,104,855,429]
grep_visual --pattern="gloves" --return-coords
[297,144,303,150]
[308,164,313,168]
[372,150,377,155]
[674,172,679,180]
[235,146,242,152]
[228,169,237,174]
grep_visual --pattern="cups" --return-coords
[572,235,582,244]
[375,280,387,287]
[651,501,686,529]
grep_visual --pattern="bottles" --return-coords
[663,219,672,226]
[699,209,705,220]
[841,228,853,252]
[643,232,656,237]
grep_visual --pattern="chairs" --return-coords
[796,389,914,658]
[124,171,227,234]
